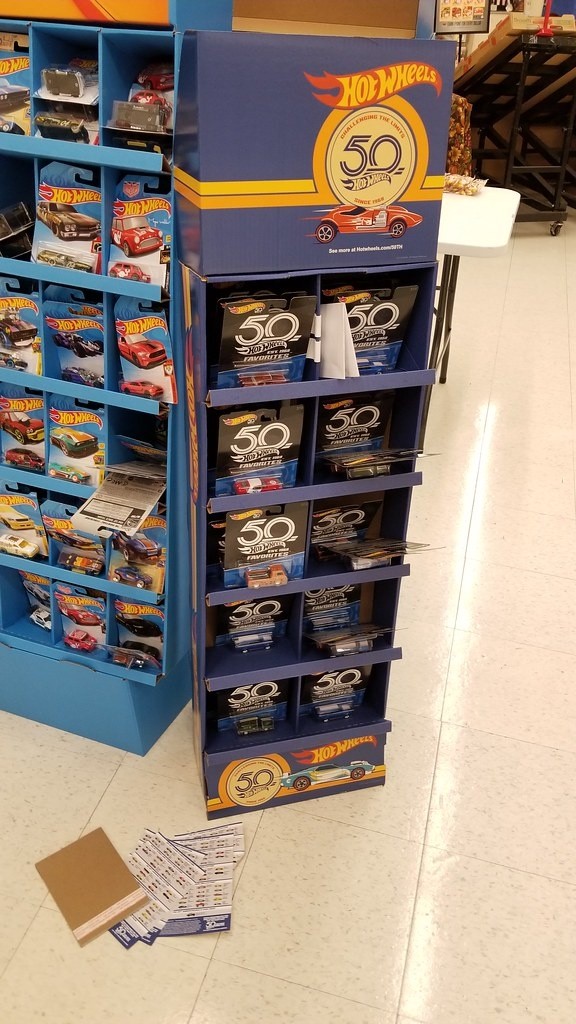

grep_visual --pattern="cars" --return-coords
[229,354,394,736]
[1,501,162,562]
[0,408,99,456]
[33,251,155,285]
[135,64,175,91]
[0,534,152,589]
[35,194,163,258]
[0,67,167,147]
[0,308,173,370]
[29,608,159,669]
[22,577,162,638]
[0,354,165,398]
[4,447,92,484]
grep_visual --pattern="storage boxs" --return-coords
[161,28,461,288]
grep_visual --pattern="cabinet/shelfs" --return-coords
[166,258,453,823]
[0,0,238,760]
